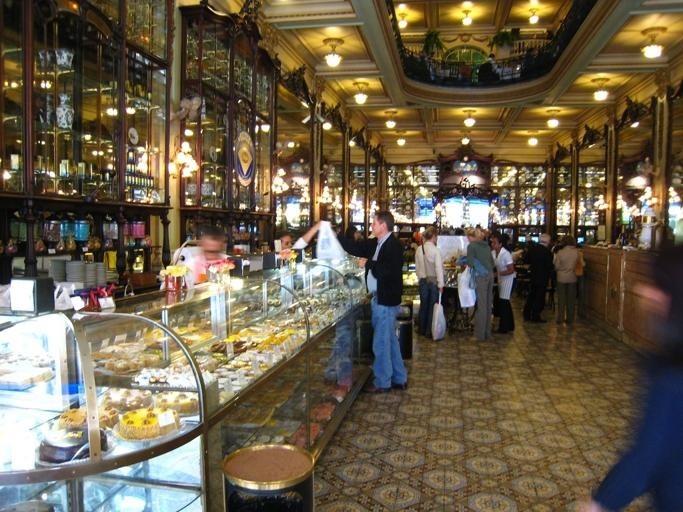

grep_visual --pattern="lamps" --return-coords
[322,36,408,147]
[456,105,481,147]
[527,25,667,145]
[389,5,546,30]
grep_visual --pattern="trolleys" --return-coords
[441,263,478,336]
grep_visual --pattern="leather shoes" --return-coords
[524,318,547,323]
[363,383,407,393]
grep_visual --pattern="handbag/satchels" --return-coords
[423,256,436,283]
[575,254,583,276]
[432,303,447,340]
[456,256,489,277]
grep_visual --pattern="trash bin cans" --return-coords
[395,304,413,360]
[222,444,314,512]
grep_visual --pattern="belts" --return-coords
[370,292,377,299]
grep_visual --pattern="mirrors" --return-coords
[551,75,682,248]
[275,66,387,237]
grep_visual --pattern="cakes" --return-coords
[39,326,307,468]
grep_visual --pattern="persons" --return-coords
[333,209,408,394]
[569,238,682,511]
[462,227,495,343]
[178,223,230,285]
[322,305,357,394]
[344,226,362,240]
[411,220,563,318]
[276,219,325,251]
[522,233,553,323]
[414,225,444,339]
[487,232,517,334]
[553,234,586,326]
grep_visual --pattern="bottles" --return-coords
[2,145,154,201]
[205,259,231,283]
[165,266,185,291]
[289,249,297,273]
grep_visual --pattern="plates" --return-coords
[65,261,107,289]
[49,259,66,281]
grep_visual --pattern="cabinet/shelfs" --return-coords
[0,252,372,512]
[0,1,279,308]
